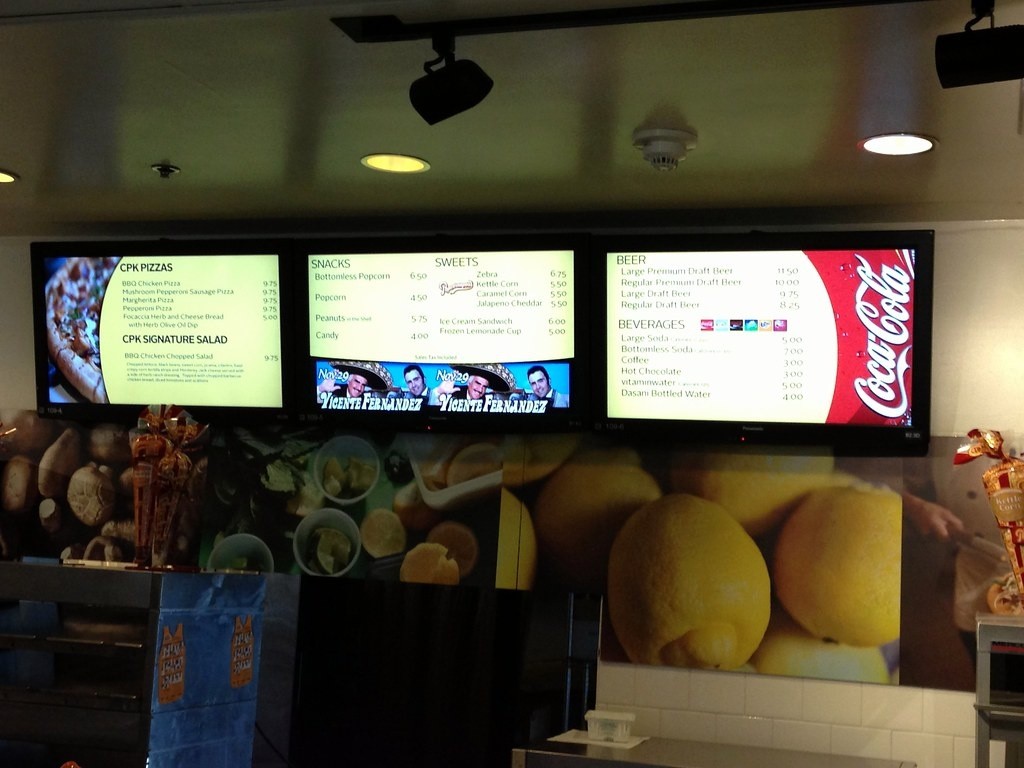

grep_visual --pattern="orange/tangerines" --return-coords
[355,433,503,584]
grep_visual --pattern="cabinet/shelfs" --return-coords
[0,559,267,768]
[972,614,1024,768]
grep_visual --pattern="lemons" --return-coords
[494,433,903,686]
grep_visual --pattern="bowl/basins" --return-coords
[405,436,504,510]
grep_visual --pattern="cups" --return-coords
[312,435,380,507]
[294,508,363,577]
[204,534,276,573]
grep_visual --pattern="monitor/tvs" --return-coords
[30,226,934,459]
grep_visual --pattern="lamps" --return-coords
[934,0,1024,88]
[409,32,494,125]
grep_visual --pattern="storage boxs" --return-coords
[584,709,635,742]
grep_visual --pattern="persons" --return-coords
[331,366,567,409]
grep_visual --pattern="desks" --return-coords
[511,730,917,768]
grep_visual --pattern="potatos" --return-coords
[0,409,135,564]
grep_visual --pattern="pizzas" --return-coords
[44,256,121,404]
